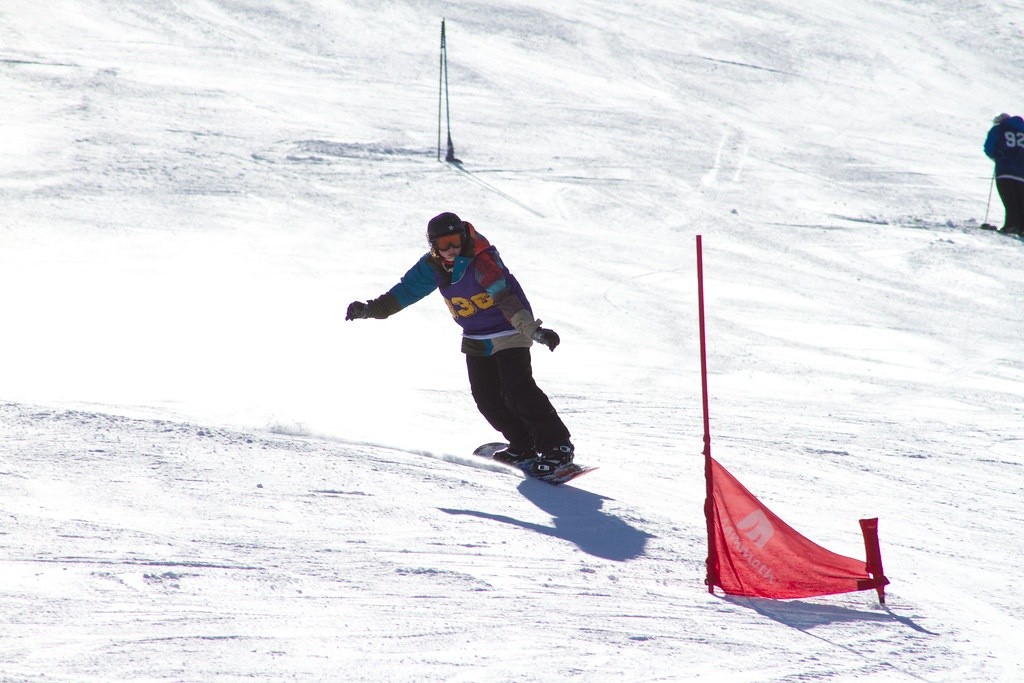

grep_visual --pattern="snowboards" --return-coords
[473,442,600,485]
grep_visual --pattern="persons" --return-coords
[983,113,1024,237]
[344,212,575,475]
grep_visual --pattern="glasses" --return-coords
[433,233,462,251]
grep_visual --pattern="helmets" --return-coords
[427,212,466,238]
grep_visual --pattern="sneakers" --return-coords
[491,438,537,465]
[999,222,1023,233]
[531,439,575,476]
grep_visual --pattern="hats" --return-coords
[997,113,1010,122]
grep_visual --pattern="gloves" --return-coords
[530,327,560,352]
[345,301,373,321]
